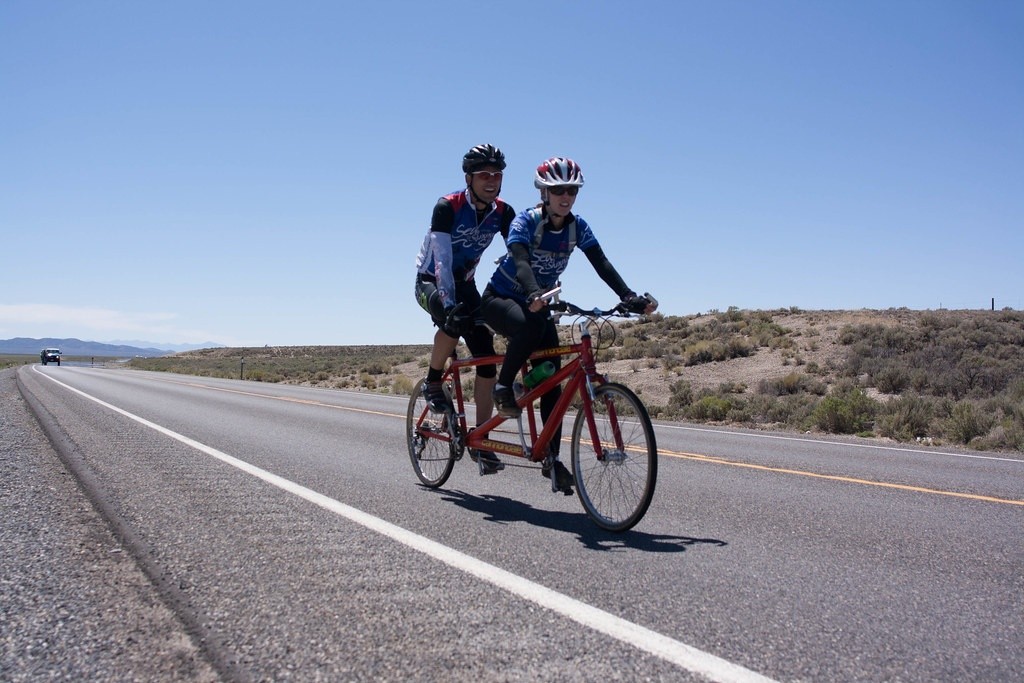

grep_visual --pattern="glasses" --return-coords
[548,186,578,196]
[473,171,502,181]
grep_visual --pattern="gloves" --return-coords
[445,313,461,333]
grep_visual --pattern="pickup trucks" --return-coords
[41,348,62,367]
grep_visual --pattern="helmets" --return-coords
[534,157,585,188]
[462,143,506,172]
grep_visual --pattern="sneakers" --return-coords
[470,447,504,471]
[491,382,522,417]
[543,460,574,485]
[421,379,450,413]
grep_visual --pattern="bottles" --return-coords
[513,382,524,400]
[523,361,555,388]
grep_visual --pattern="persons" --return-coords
[478,156,655,486]
[415,144,515,471]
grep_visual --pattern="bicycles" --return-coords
[406,285,658,533]
